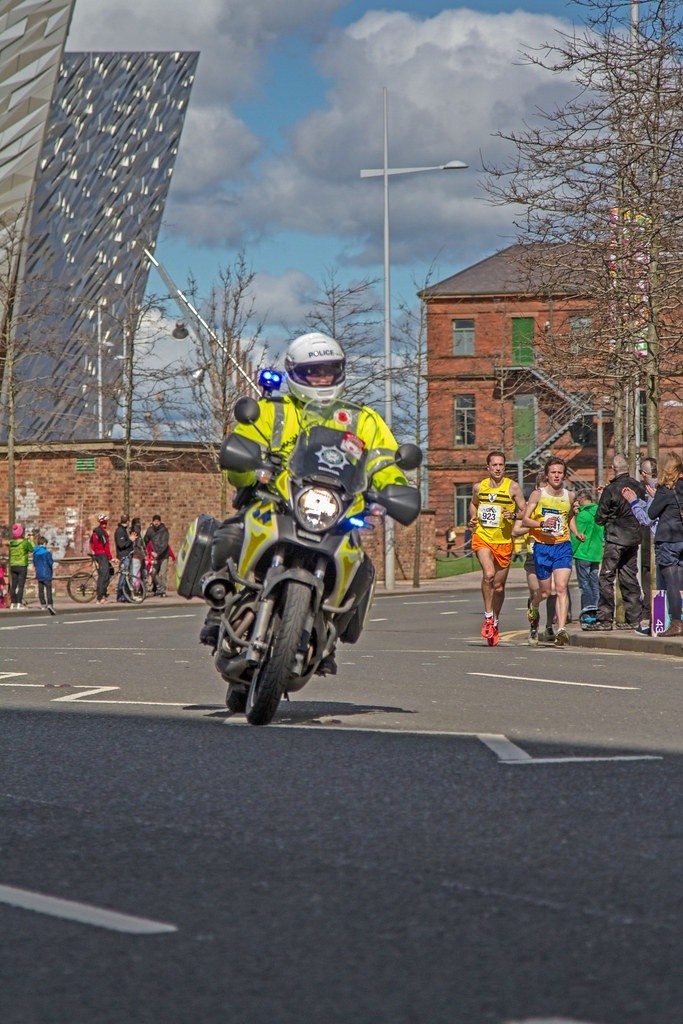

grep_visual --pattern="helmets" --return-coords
[285,333,346,408]
[97,514,109,522]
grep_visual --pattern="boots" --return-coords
[657,620,683,636]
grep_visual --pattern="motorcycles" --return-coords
[175,365,424,726]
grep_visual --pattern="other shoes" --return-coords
[10,603,17,609]
[307,645,337,675]
[42,604,47,610]
[96,600,110,605]
[47,605,56,615]
[634,627,651,636]
[17,603,25,609]
[199,620,220,644]
[616,621,640,630]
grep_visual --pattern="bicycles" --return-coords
[66,549,147,604]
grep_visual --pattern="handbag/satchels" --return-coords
[651,590,683,637]
[448,530,456,542]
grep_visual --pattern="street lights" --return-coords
[360,87,471,590]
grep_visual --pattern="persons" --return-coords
[90,513,115,605]
[33,537,56,616]
[466,450,683,649]
[445,524,458,557]
[116,514,171,603]
[9,522,36,610]
[198,332,409,676]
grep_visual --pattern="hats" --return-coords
[153,515,161,521]
[12,524,23,536]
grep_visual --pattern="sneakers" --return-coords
[527,599,613,648]
[481,616,494,638]
[488,624,499,646]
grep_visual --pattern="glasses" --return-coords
[301,365,342,377]
[639,470,646,475]
